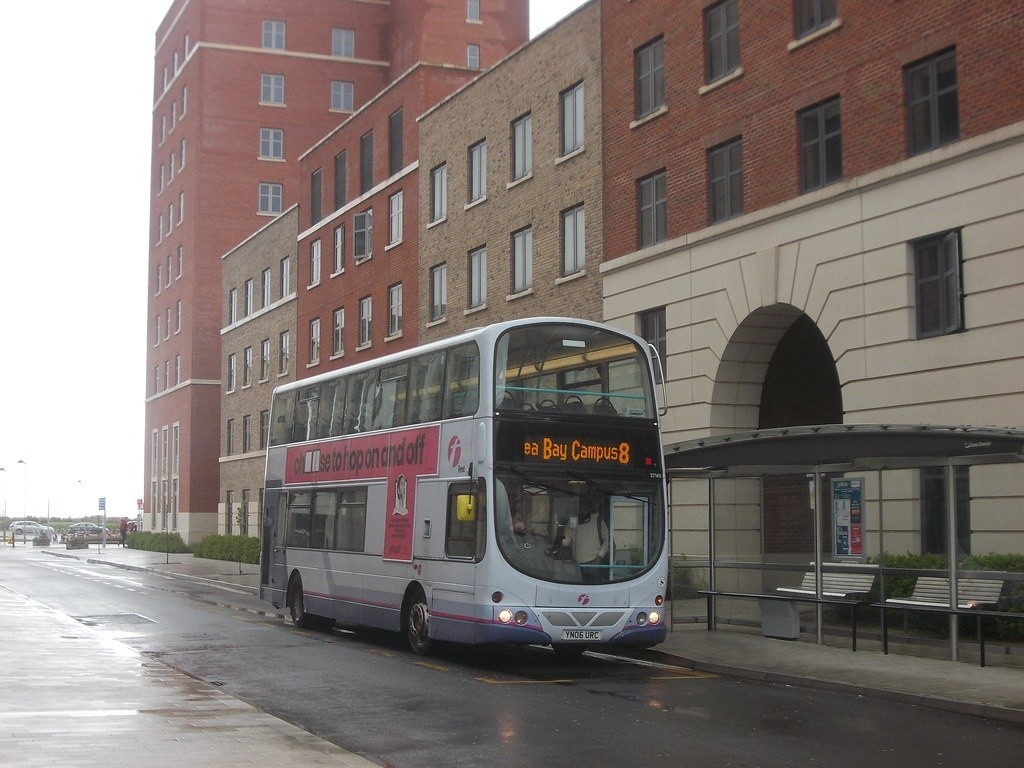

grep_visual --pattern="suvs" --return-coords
[9,521,55,536]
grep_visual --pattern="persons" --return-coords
[118,516,131,547]
[502,509,525,535]
[555,499,609,585]
[392,475,408,515]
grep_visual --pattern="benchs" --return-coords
[776,561,880,625]
[885,570,1005,642]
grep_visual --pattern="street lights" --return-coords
[0,468,6,541]
[17,460,26,544]
[78,480,100,554]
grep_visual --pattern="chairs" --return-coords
[491,390,620,412]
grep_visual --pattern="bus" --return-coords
[260,316,675,660]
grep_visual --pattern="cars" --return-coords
[69,522,109,535]
[126,521,144,531]
